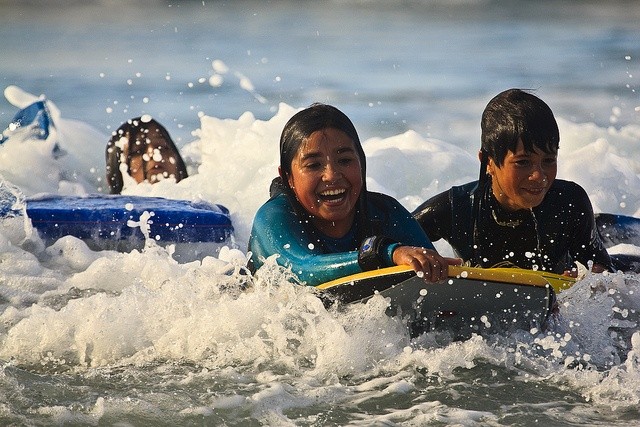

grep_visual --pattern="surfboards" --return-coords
[0,195,235,244]
[313,263,579,336]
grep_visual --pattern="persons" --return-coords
[248,100,464,285]
[409,90,617,276]
[106,116,190,194]
